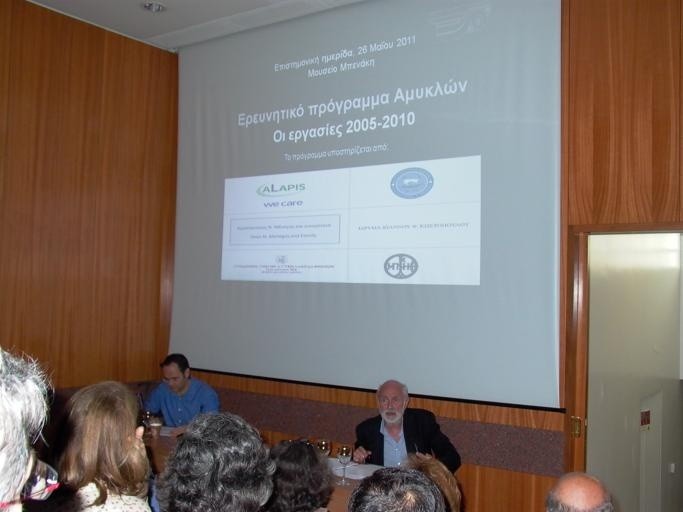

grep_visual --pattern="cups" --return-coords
[149,417,163,439]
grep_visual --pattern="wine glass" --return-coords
[141,408,152,433]
[316,438,352,486]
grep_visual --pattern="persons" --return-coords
[143,353,219,436]
[0,344,55,511]
[352,380,462,475]
[347,449,461,512]
[259,440,336,511]
[546,471,614,512]
[153,411,277,511]
[57,381,152,511]
